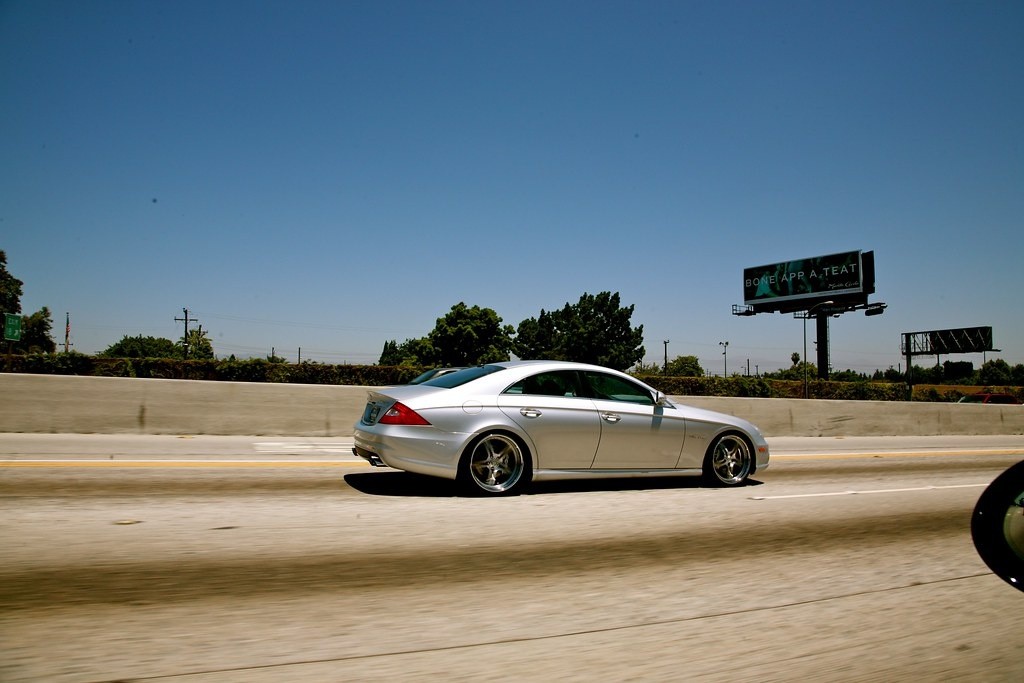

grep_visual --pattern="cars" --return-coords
[956,392,1022,403]
[351,360,771,497]
[412,366,469,386]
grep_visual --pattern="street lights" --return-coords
[719,341,729,378]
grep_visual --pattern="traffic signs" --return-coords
[4,313,21,341]
